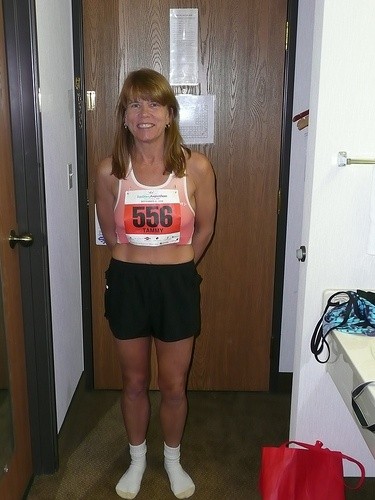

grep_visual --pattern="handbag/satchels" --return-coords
[259,441,365,500]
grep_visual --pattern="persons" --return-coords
[95,68,217,500]
